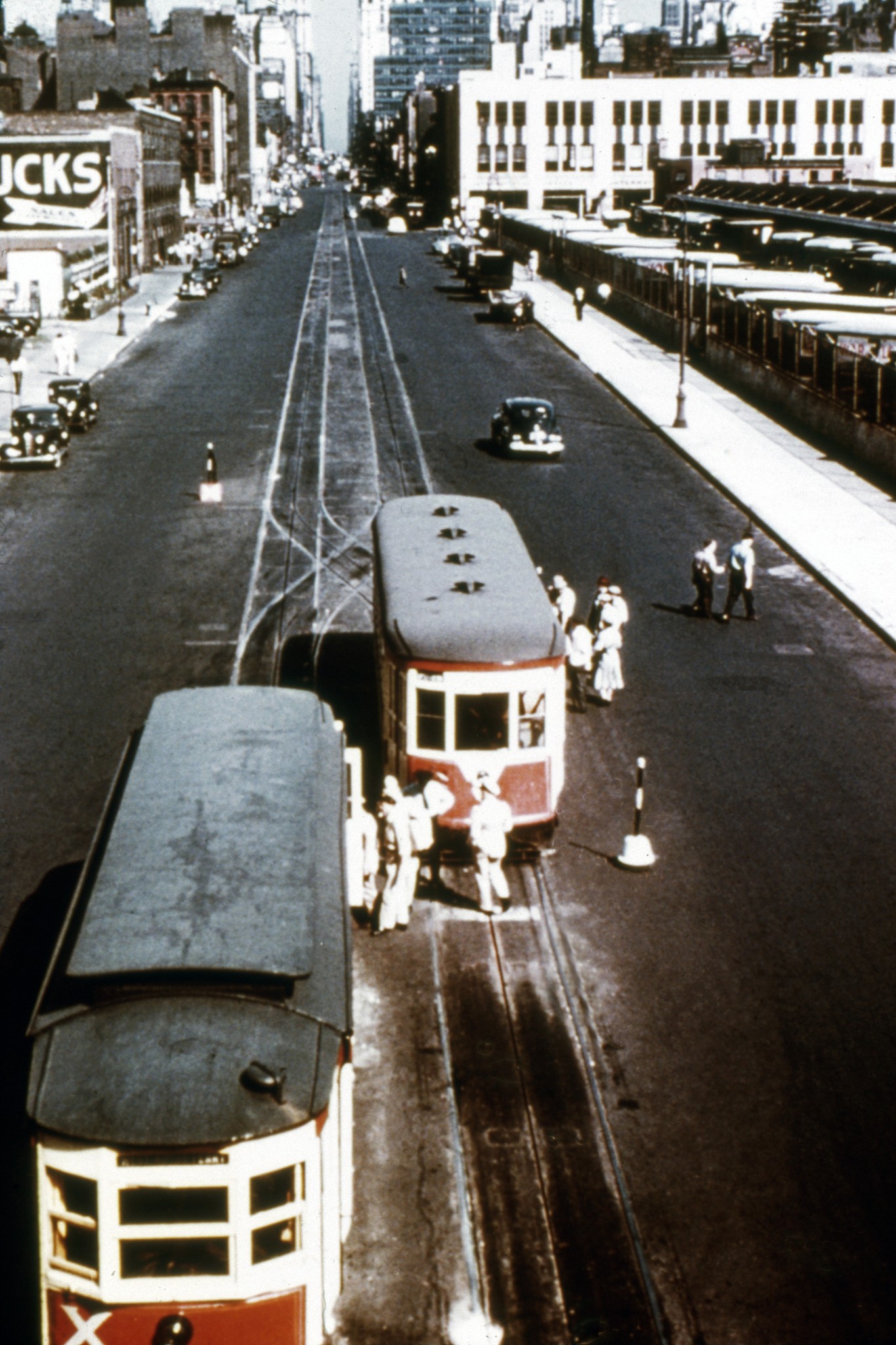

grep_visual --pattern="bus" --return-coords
[23,686,355,1345]
[371,495,568,861]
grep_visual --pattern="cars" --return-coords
[0,376,99,468]
[491,397,566,461]
[178,184,296,293]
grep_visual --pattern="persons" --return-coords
[11,357,27,395]
[398,265,409,289]
[53,334,77,375]
[404,770,455,888]
[568,617,593,713]
[167,241,194,266]
[686,539,727,619]
[548,575,577,633]
[595,615,626,706]
[723,532,759,622]
[589,577,629,637]
[574,288,586,321]
[154,252,164,268]
[349,770,422,936]
[529,250,539,280]
[466,781,514,915]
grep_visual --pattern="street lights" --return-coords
[658,196,692,428]
